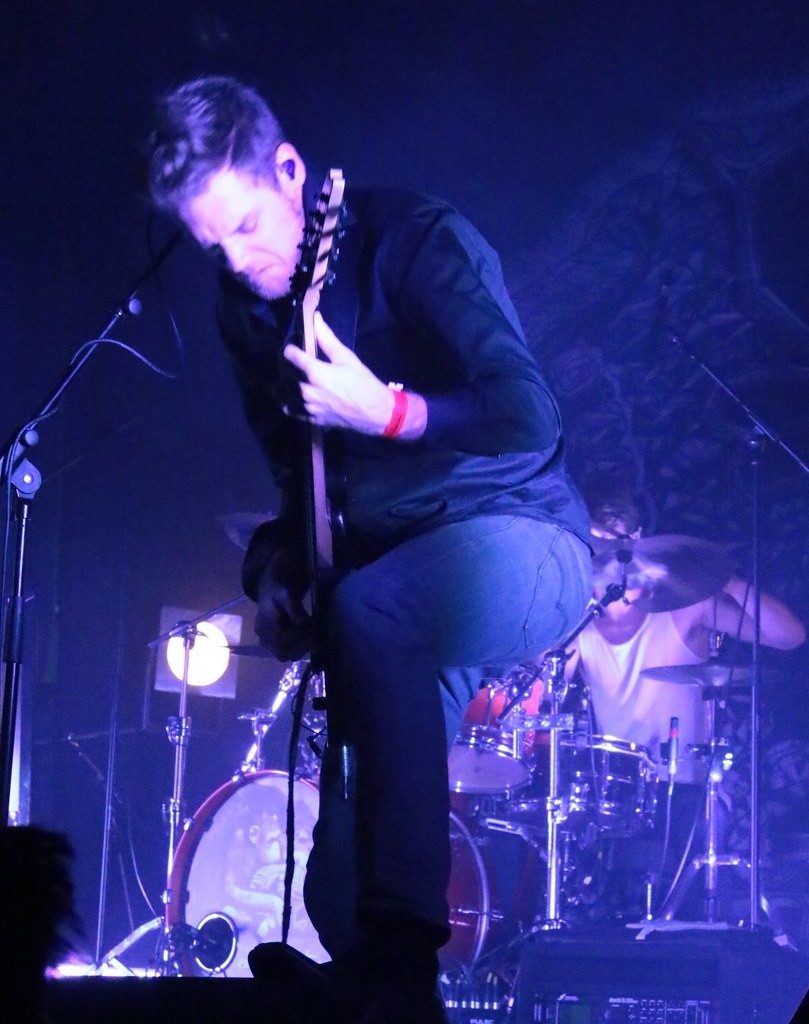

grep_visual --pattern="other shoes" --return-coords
[246,940,364,994]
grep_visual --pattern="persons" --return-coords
[530,490,805,998]
[136,80,602,1024]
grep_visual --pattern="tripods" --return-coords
[636,630,787,943]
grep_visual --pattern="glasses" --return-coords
[592,537,635,566]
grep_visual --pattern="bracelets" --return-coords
[379,382,409,440]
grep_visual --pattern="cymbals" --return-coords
[639,652,771,689]
[592,534,740,615]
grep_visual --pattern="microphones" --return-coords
[667,716,679,795]
[178,922,227,950]
[652,269,673,331]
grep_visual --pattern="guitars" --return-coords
[251,159,352,663]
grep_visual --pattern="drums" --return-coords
[501,711,576,830]
[558,733,654,840]
[160,764,493,989]
[444,661,544,796]
